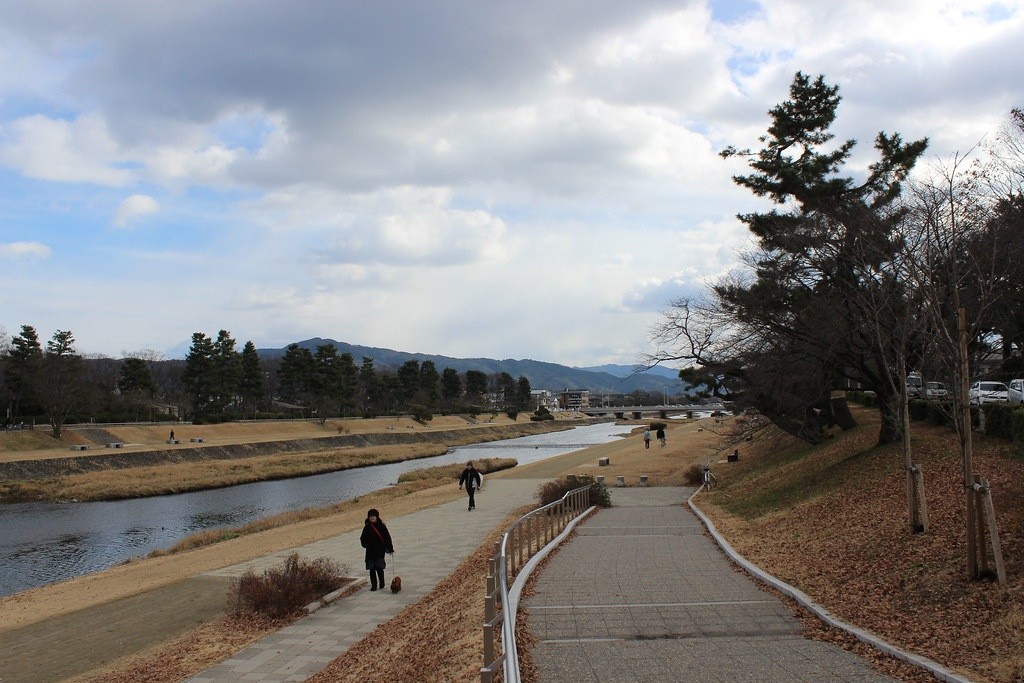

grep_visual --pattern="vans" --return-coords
[905,375,923,398]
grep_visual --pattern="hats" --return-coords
[467,462,473,466]
[368,509,379,519]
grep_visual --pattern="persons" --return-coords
[644,429,652,449]
[657,427,666,448]
[459,461,481,511]
[170,429,175,440]
[360,509,394,591]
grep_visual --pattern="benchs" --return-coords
[191,438,203,443]
[71,445,88,451]
[746,435,752,441]
[166,439,182,444]
[599,457,610,465]
[727,448,738,462]
[106,442,123,449]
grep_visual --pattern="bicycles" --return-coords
[701,459,717,491]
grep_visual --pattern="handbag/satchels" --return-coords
[644,437,647,441]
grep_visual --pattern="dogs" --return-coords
[390,577,401,595]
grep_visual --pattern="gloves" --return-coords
[477,487,480,491]
[458,486,462,490]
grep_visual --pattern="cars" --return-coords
[969,380,1009,406]
[1006,377,1024,406]
[925,382,949,401]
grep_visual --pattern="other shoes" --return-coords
[468,506,472,511]
[380,579,384,589]
[370,586,377,591]
